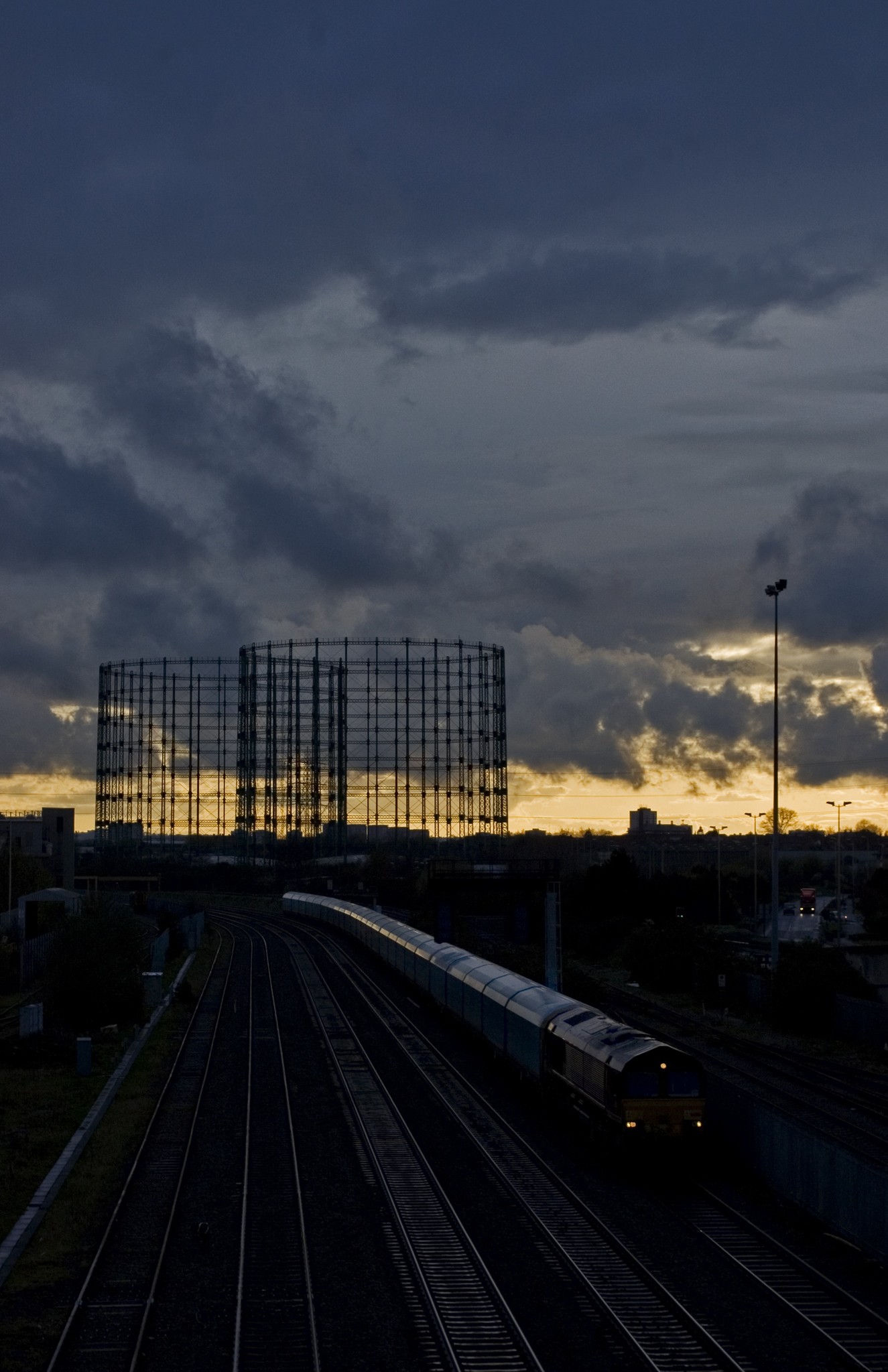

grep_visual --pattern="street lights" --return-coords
[764,577,791,981]
[709,824,730,925]
[828,800,860,947]
[743,809,768,916]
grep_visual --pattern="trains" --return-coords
[279,891,705,1146]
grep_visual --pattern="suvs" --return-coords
[784,903,795,915]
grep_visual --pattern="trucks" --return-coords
[797,887,820,914]
